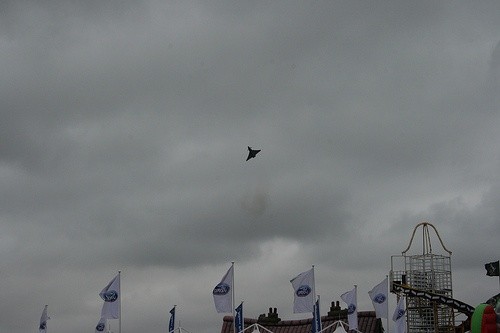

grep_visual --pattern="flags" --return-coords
[368,278,387,320]
[340,288,358,330]
[289,267,313,313]
[39,307,50,333]
[391,297,406,333]
[485,261,499,276]
[94,273,119,333]
[213,265,234,313]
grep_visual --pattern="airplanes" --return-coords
[245,145,261,161]
